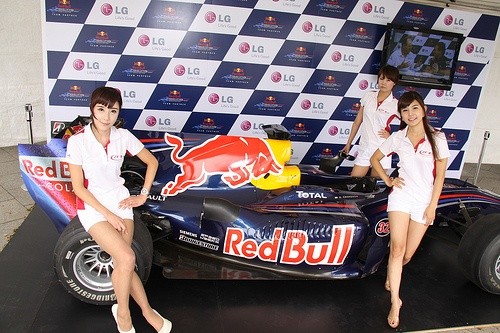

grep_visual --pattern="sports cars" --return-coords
[17,125,500,306]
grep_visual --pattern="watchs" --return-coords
[141,188,149,195]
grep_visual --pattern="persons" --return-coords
[66,88,172,333]
[386,36,424,71]
[340,65,402,176]
[421,42,451,76]
[370,91,452,328]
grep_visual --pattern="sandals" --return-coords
[388,300,402,328]
[385,280,391,291]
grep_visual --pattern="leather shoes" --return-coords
[151,309,172,333]
[112,304,135,333]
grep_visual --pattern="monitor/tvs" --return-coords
[381,22,463,91]
